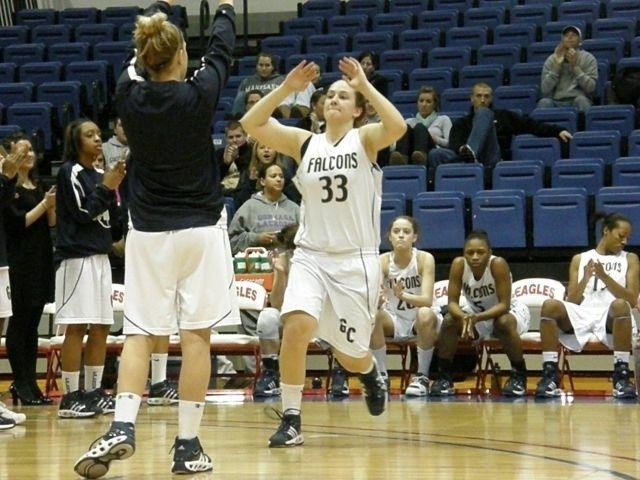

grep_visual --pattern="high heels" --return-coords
[9,381,54,405]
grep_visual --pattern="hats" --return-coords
[562,25,580,37]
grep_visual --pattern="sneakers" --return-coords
[171,435,212,474]
[431,379,456,396]
[73,423,135,479]
[389,151,405,165]
[57,386,117,419]
[332,367,350,396]
[412,150,425,165]
[147,379,178,404]
[406,374,429,395]
[502,374,526,395]
[459,144,477,164]
[268,415,302,446]
[536,367,564,397]
[613,366,636,399]
[0,400,25,430]
[359,358,388,416]
[253,373,281,397]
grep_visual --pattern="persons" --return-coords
[215,55,397,398]
[389,82,575,196]
[370,211,639,399]
[240,57,407,446]
[536,25,598,131]
[75,0,235,479]
[1,117,179,429]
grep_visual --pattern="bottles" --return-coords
[312,376,322,401]
[490,363,502,390]
[232,251,273,274]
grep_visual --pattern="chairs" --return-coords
[0,0,640,398]
